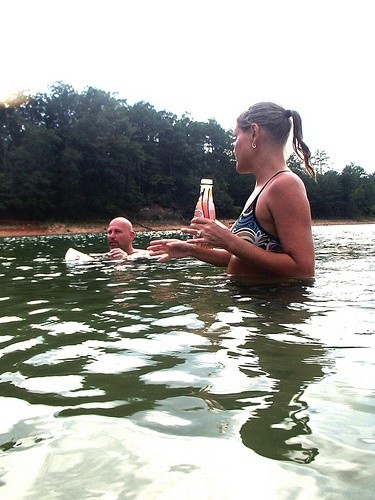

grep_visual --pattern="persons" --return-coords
[105,217,152,261]
[147,101,318,282]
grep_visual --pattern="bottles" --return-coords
[192,179,216,249]
